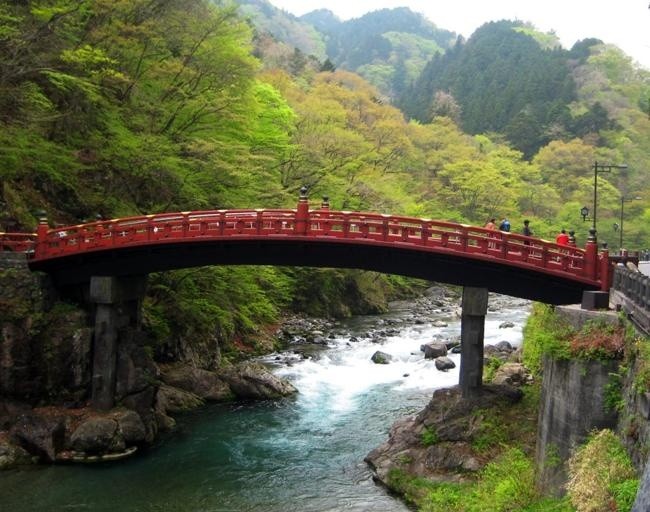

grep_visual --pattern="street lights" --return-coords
[619,195,642,248]
[592,161,627,228]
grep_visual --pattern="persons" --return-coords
[611,258,625,270]
[498,216,510,232]
[621,256,639,272]
[568,230,576,263]
[556,229,570,263]
[523,219,534,243]
[487,218,495,247]
